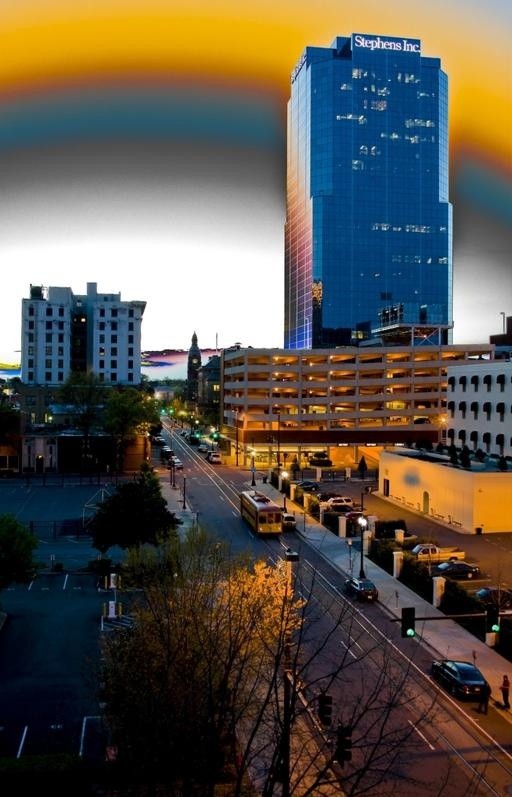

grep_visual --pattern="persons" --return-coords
[500,676,510,708]
[479,681,490,715]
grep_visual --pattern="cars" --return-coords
[430,657,490,703]
[329,420,349,428]
[197,443,207,452]
[292,480,372,536]
[281,511,297,530]
[153,432,183,471]
[414,417,430,423]
[177,429,204,445]
[470,585,512,610]
[431,560,482,579]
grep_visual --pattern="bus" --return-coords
[239,489,284,537]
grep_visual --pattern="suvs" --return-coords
[344,574,380,602]
[208,452,222,463]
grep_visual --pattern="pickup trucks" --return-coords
[404,542,467,566]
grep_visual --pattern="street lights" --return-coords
[188,508,224,571]
[195,417,199,429]
[189,414,193,435]
[177,409,186,428]
[180,473,189,509]
[354,516,370,577]
[249,448,258,486]
[231,408,241,467]
[169,404,173,423]
[346,537,354,571]
[282,492,287,510]
[281,544,303,796]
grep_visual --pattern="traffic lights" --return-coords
[401,606,415,636]
[209,433,219,439]
[338,724,355,768]
[318,692,335,725]
[484,603,499,632]
